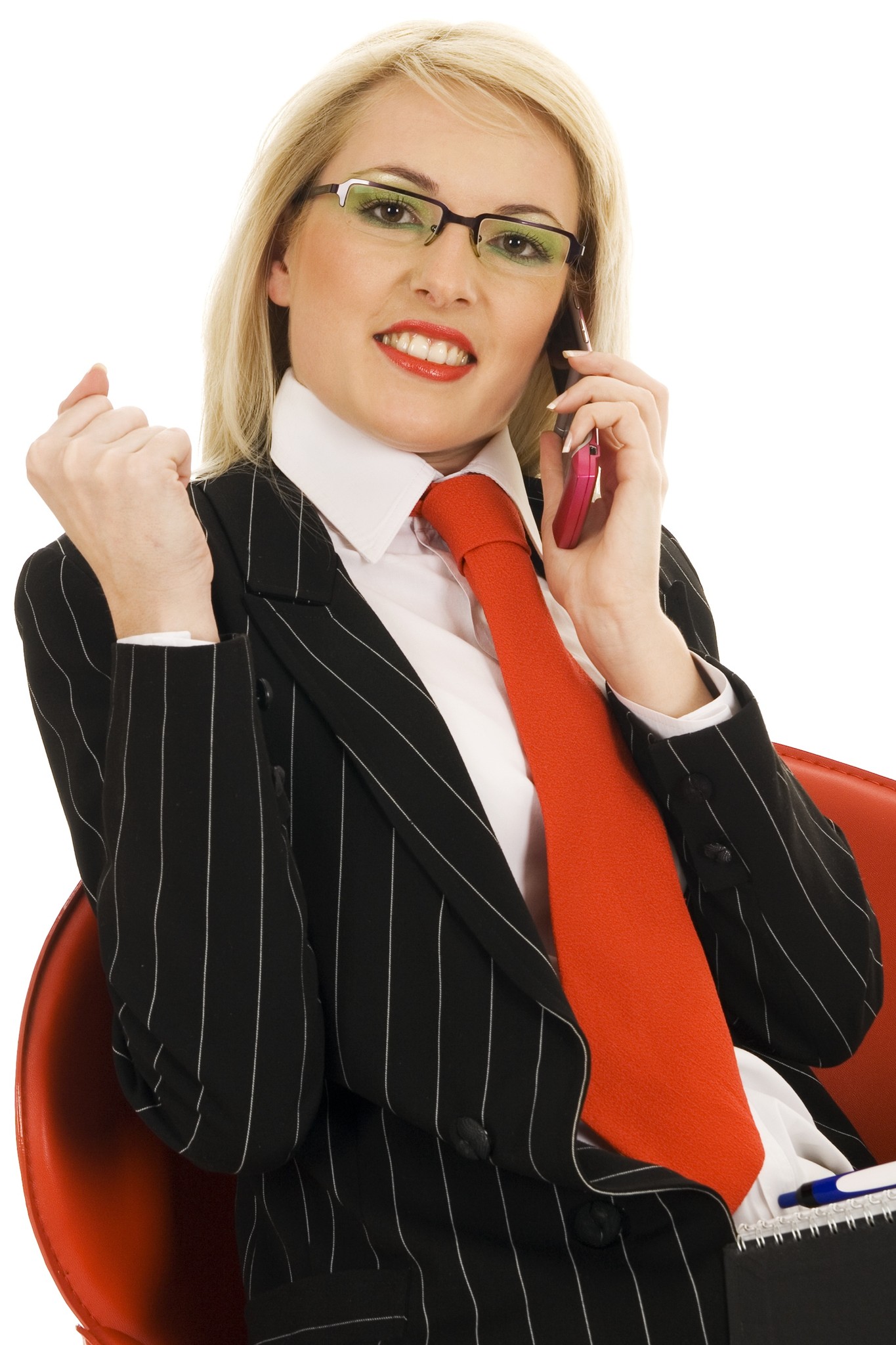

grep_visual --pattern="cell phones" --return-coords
[543,285,601,551]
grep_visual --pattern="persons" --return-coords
[16,14,885,1344]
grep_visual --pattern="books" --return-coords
[724,1186,896,1344]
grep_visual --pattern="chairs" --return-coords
[16,739,896,1345]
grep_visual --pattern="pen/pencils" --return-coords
[778,1161,896,1208]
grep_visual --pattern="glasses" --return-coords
[304,177,586,276]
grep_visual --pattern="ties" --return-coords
[407,473,765,1198]
